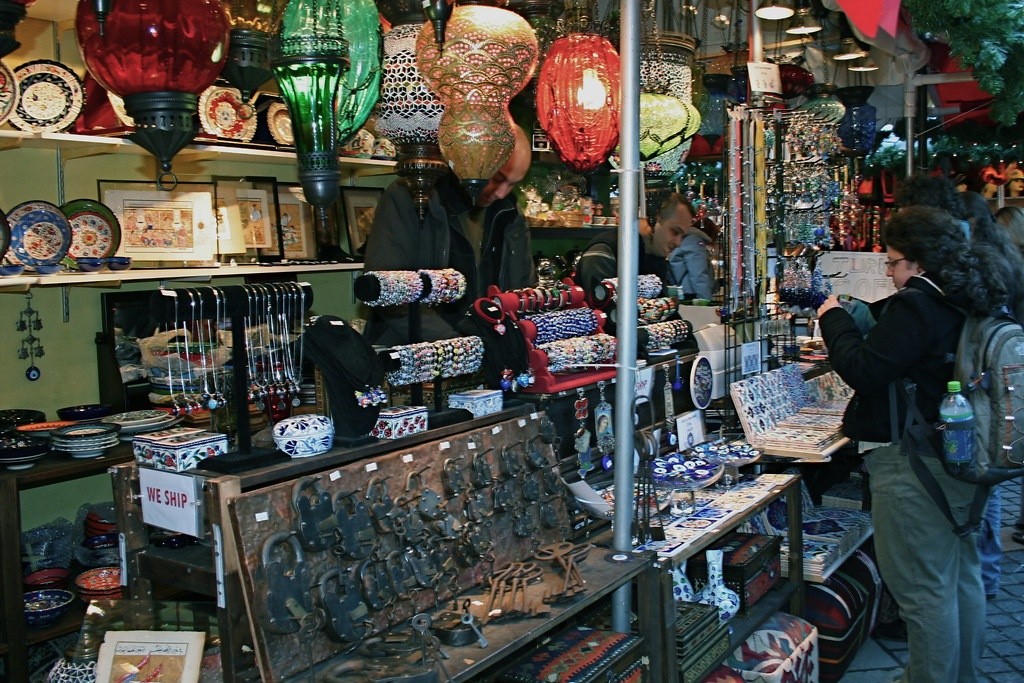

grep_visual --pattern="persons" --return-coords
[1005,169,1024,198]
[363,118,537,389]
[668,218,719,301]
[575,193,696,333]
[894,172,1024,601]
[817,205,987,683]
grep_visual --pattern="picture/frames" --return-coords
[97,178,221,271]
[274,181,319,261]
[212,176,287,265]
[340,184,390,261]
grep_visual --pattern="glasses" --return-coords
[884,255,908,270]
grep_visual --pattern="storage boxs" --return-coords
[494,625,649,683]
[686,533,785,612]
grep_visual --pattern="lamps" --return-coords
[848,57,880,72]
[74,0,710,225]
[832,29,868,61]
[753,0,796,19]
[786,3,824,34]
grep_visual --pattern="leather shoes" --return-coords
[1016,522,1024,529]
[1012,532,1024,545]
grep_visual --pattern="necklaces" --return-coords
[165,281,300,416]
[475,298,506,335]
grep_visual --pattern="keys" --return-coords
[297,438,561,683]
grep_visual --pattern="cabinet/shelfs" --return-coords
[0,0,395,287]
[1,430,803,682]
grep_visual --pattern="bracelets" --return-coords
[637,320,688,351]
[419,268,467,307]
[504,283,572,314]
[602,274,663,303]
[363,271,423,307]
[637,297,676,326]
[384,336,484,387]
[536,334,616,373]
[519,306,598,344]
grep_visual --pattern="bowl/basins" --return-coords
[56,404,114,421]
[1,265,24,275]
[20,506,198,629]
[33,264,65,274]
[76,256,132,271]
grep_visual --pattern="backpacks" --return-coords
[882,287,1024,488]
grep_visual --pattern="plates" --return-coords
[58,199,122,271]
[0,58,20,127]
[0,208,11,264]
[0,409,52,464]
[340,128,396,161]
[48,409,184,459]
[4,200,72,272]
[267,102,296,146]
[197,83,258,143]
[10,59,87,134]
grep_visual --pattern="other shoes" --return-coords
[873,616,908,642]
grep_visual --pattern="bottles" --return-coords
[939,380,975,466]
[671,549,740,635]
